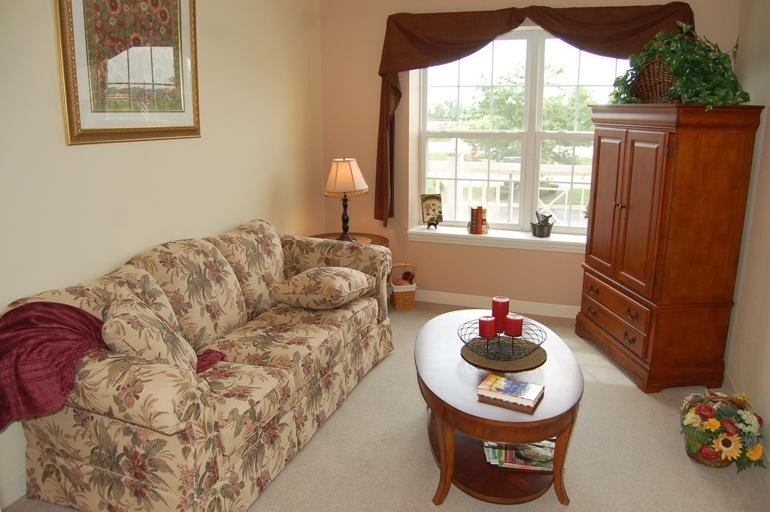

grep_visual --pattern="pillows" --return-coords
[101,286,197,375]
[271,265,376,309]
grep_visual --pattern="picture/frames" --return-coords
[53,1,201,145]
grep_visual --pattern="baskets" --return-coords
[459,314,545,359]
[631,50,681,105]
[682,396,749,468]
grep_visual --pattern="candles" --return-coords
[504,315,522,335]
[480,316,496,338]
[492,296,509,332]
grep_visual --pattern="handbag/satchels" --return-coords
[388,262,416,310]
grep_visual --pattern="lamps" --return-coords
[324,157,369,243]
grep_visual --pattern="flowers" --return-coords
[680,391,766,475]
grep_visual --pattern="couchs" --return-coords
[0,218,394,512]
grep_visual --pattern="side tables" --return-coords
[312,230,390,253]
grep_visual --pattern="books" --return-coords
[481,436,556,472]
[477,375,544,408]
[470,203,487,234]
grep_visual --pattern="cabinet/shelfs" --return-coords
[575,104,765,393]
